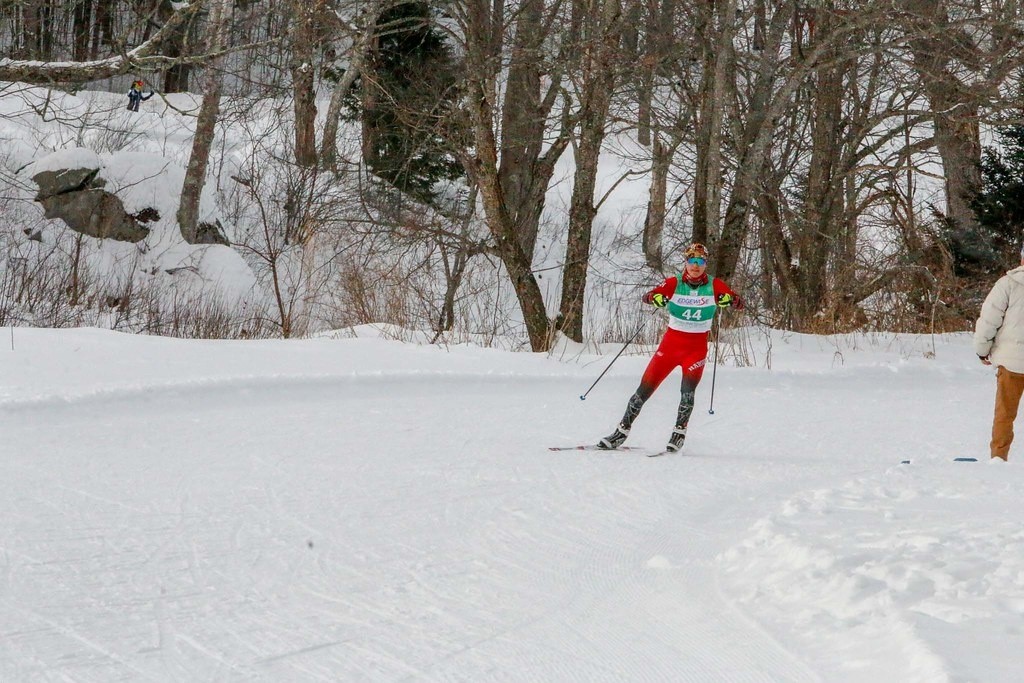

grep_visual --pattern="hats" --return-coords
[684,242,709,263]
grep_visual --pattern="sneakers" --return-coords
[600,421,631,448]
[667,425,687,452]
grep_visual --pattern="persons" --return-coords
[599,244,746,453]
[127,78,143,112]
[974,247,1024,462]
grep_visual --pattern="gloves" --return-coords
[652,293,668,307]
[718,293,735,308]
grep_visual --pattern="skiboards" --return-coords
[548,446,667,458]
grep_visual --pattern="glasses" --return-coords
[687,257,705,266]
